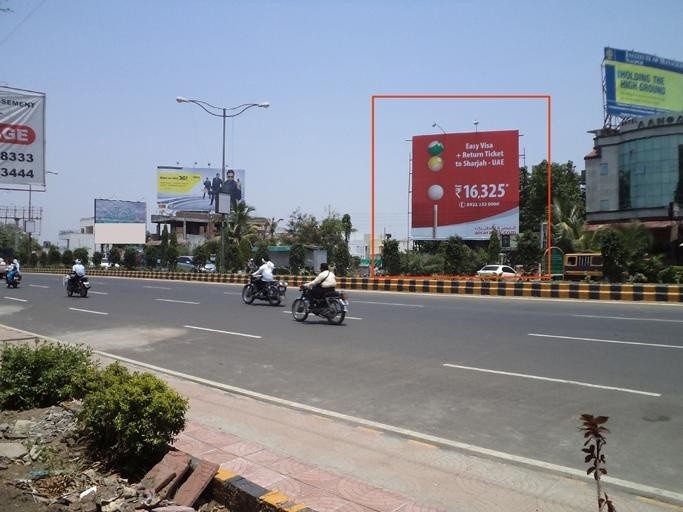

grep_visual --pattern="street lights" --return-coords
[177,99,270,271]
[28,171,58,267]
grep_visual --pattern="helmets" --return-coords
[75,258,81,264]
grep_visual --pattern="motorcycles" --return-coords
[242,274,288,306]
[5,270,22,288]
[292,285,349,325]
[63,272,90,297]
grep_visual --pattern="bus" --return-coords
[564,251,629,283]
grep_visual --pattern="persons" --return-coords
[303,262,337,309]
[219,170,241,213]
[250,255,274,296]
[209,173,224,205]
[69,259,85,291]
[6,259,19,288]
[202,177,212,200]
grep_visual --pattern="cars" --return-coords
[477,266,523,281]
[91,258,120,268]
[167,256,216,273]
